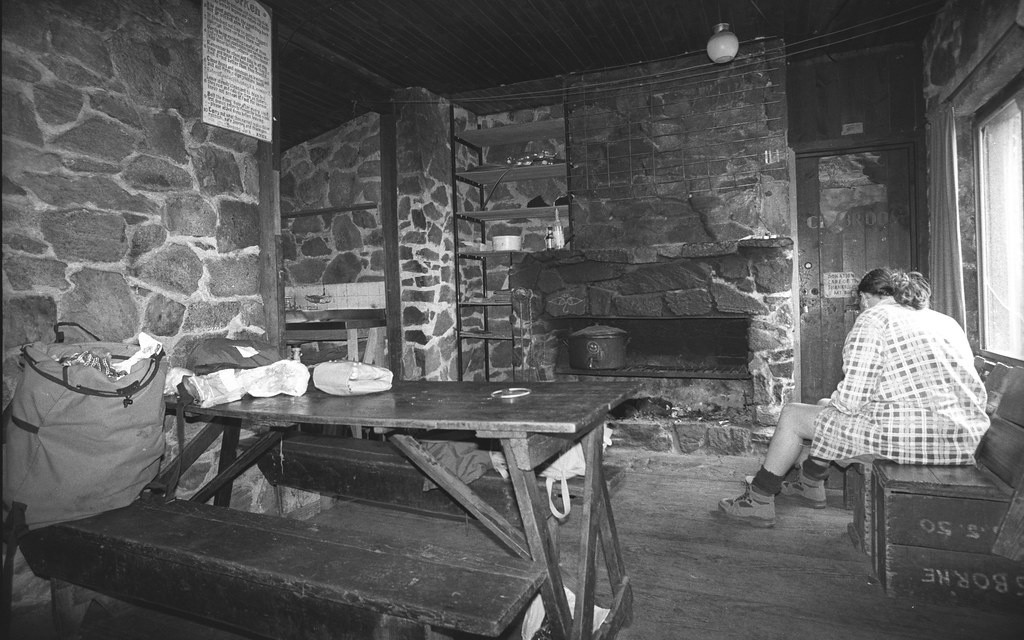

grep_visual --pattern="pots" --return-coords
[562,322,632,371]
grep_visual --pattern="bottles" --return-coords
[546,227,552,249]
[552,208,565,249]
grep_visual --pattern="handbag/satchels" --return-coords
[489,426,614,519]
[312,360,393,397]
[6,321,168,537]
[186,338,281,377]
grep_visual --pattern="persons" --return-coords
[717,266,992,527]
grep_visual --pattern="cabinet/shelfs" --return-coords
[447,86,574,384]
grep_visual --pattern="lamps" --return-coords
[706,0,740,62]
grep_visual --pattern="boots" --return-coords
[717,484,776,527]
[778,471,827,508]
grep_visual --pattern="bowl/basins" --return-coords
[493,236,522,251]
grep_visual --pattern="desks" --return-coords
[161,376,647,639]
[284,318,388,366]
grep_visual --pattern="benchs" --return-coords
[242,426,623,568]
[858,357,1024,613]
[47,495,548,640]
